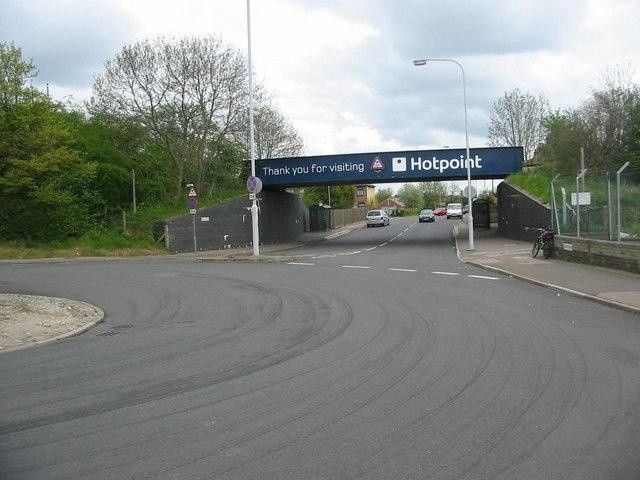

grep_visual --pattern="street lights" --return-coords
[412,56,477,252]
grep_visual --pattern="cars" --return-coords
[434,208,447,216]
[462,205,470,214]
[365,210,389,227]
[418,208,435,223]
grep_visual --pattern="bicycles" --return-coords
[529,226,556,260]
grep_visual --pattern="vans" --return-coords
[446,203,463,220]
